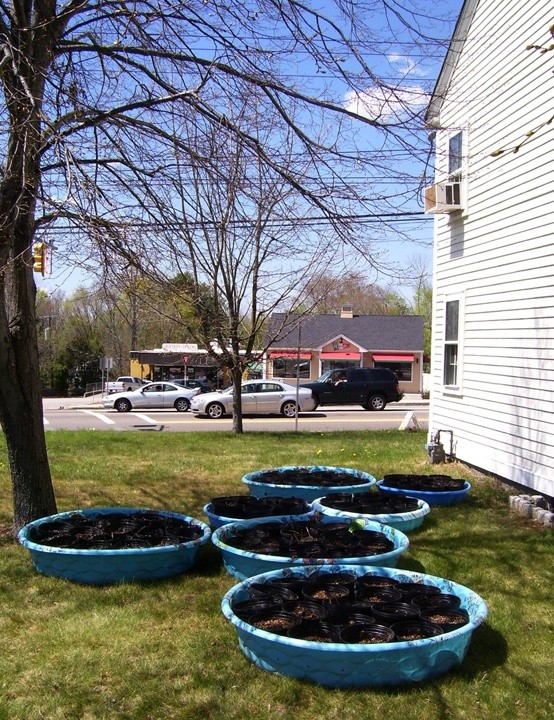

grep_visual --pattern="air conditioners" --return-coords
[423,181,466,215]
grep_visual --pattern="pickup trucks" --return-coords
[105,376,153,395]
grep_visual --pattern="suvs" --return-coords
[300,367,406,412]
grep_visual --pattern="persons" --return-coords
[332,373,350,386]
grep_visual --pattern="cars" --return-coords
[191,380,315,418]
[102,381,198,412]
